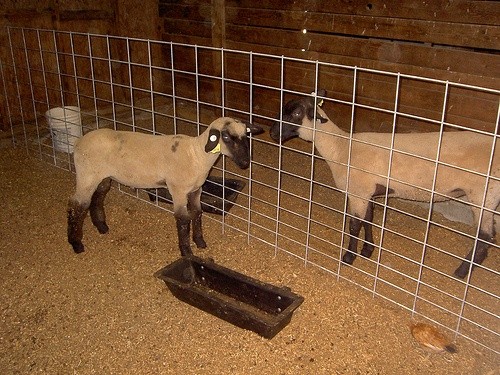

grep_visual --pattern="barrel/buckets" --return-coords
[46,106,81,154]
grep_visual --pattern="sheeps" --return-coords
[269,88,500,280]
[66,116,265,257]
